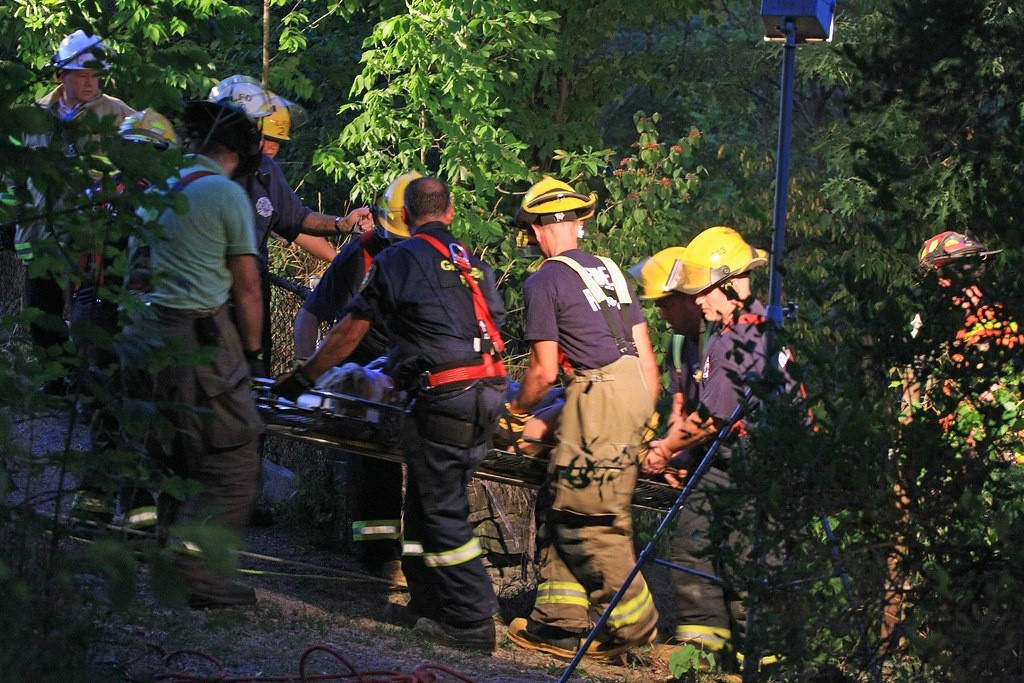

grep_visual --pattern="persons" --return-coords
[294,169,425,589]
[272,174,509,656]
[328,356,659,466]
[497,179,660,660]
[634,230,1024,683]
[17,29,374,611]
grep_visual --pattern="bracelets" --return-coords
[335,217,346,235]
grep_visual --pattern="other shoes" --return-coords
[75,512,113,530]
[361,539,403,562]
[507,617,579,659]
[580,623,658,659]
[381,600,428,629]
[126,526,154,540]
[413,617,497,650]
[187,578,258,604]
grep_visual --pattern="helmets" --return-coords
[663,226,769,295]
[627,247,687,299]
[207,73,276,118]
[256,90,308,144]
[918,231,1004,277]
[369,170,424,238]
[508,175,599,229]
[44,30,113,70]
[116,106,178,149]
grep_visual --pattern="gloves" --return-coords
[250,349,266,377]
[496,404,534,448]
[269,365,315,403]
